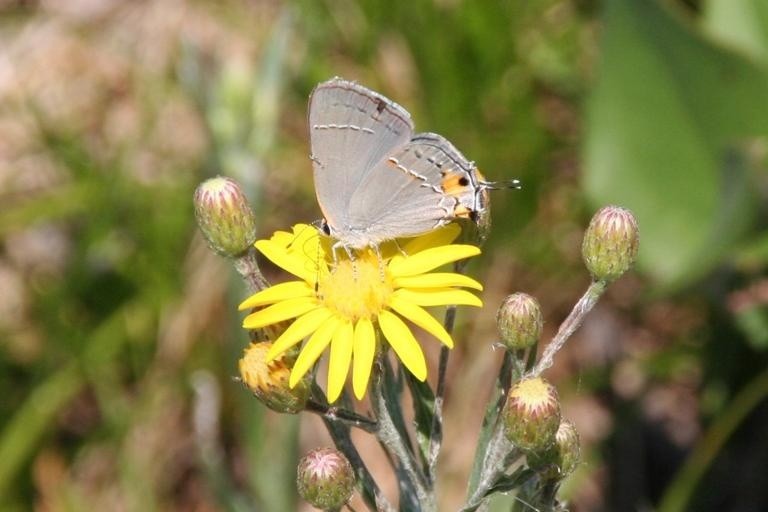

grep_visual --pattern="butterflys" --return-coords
[281,75,522,299]
